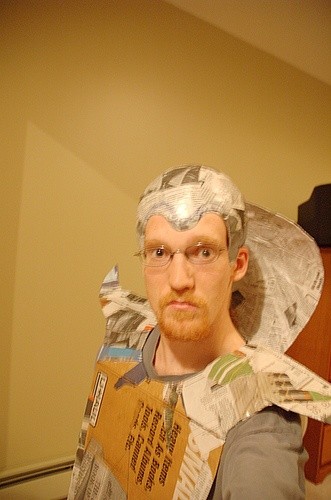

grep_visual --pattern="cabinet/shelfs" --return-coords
[284,249,331,485]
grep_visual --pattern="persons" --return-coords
[69,165,309,500]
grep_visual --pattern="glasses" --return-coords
[134,242,227,267]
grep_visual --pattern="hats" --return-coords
[137,165,247,265]
